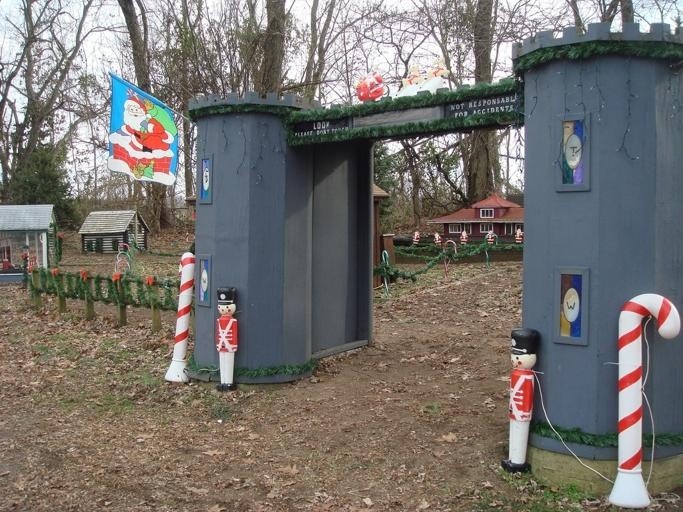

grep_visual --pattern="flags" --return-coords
[108,75,179,186]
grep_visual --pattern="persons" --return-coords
[502,327,540,472]
[215,288,238,391]
